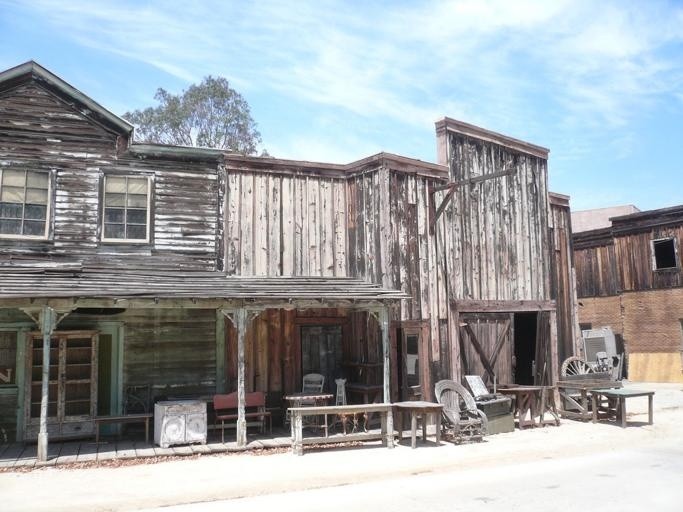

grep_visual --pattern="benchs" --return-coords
[212,390,272,442]
[90,413,150,445]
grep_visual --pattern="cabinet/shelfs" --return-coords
[153,400,206,448]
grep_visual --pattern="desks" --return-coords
[286,393,333,432]
[591,388,655,426]
[393,400,443,448]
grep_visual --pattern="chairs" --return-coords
[283,373,323,432]
[434,379,487,444]
